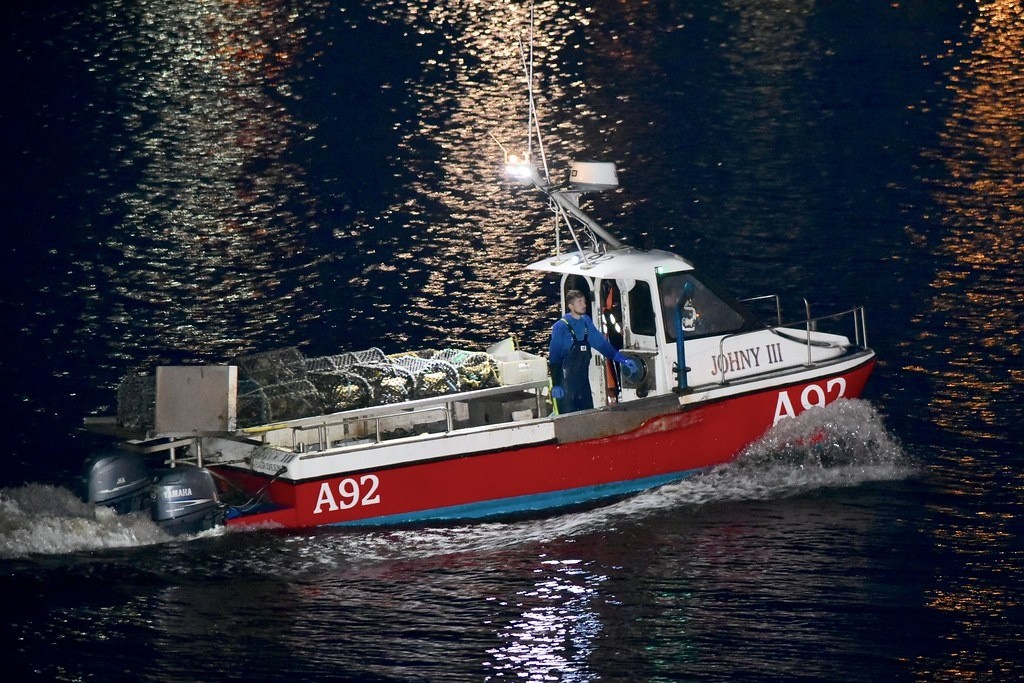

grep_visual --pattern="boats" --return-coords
[79,9,877,542]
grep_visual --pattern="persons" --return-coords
[549,289,637,414]
[639,282,681,342]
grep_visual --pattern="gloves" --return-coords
[551,386,565,399]
[625,359,639,373]
